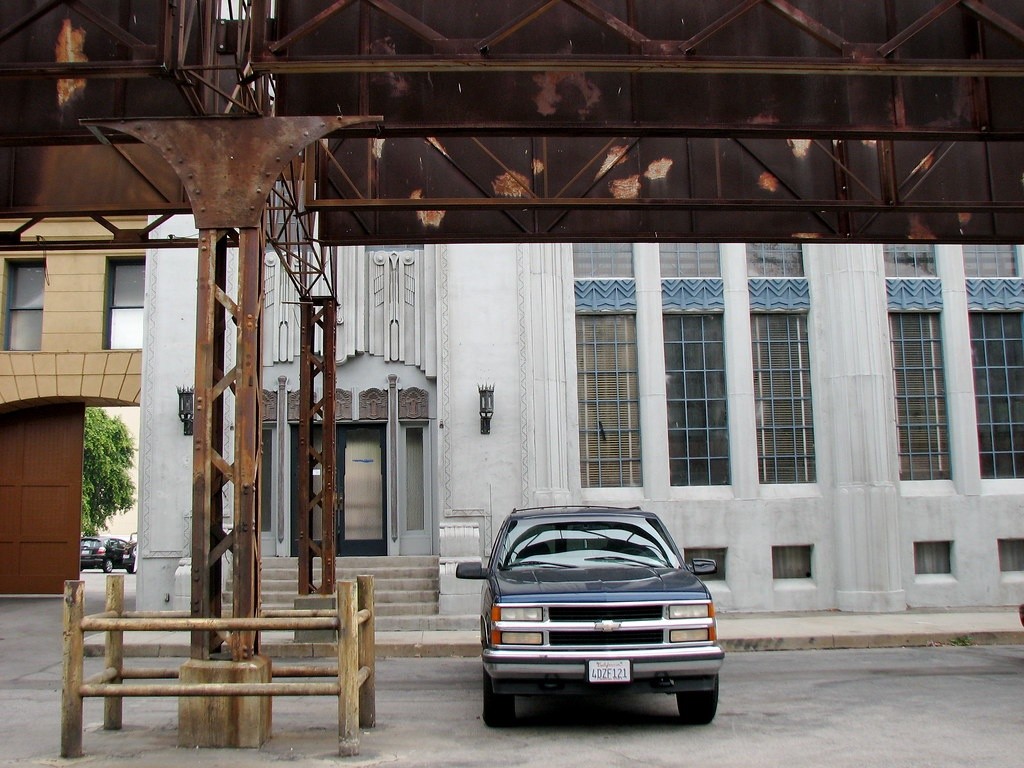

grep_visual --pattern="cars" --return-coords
[122,539,137,573]
[80,536,127,573]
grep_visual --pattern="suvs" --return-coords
[453,503,727,726]
